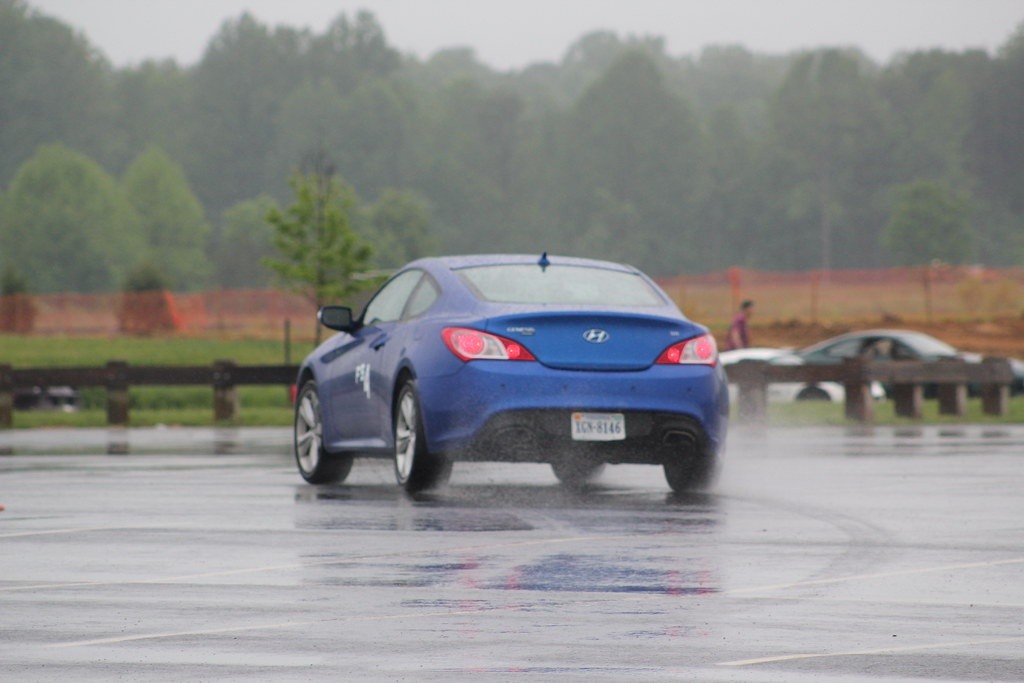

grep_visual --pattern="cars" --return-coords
[791,327,1023,403]
[291,252,731,503]
[717,346,886,405]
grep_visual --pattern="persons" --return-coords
[727,300,753,349]
